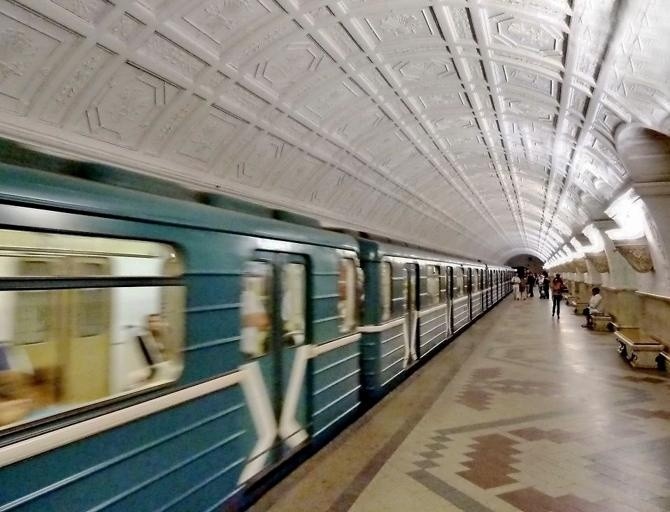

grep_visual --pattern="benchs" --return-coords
[571,298,665,370]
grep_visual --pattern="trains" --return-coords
[0,162,518,512]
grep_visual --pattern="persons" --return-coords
[146,315,164,351]
[581,288,602,328]
[0,366,63,426]
[239,273,270,360]
[550,278,563,318]
[553,274,563,284]
[511,272,550,300]
